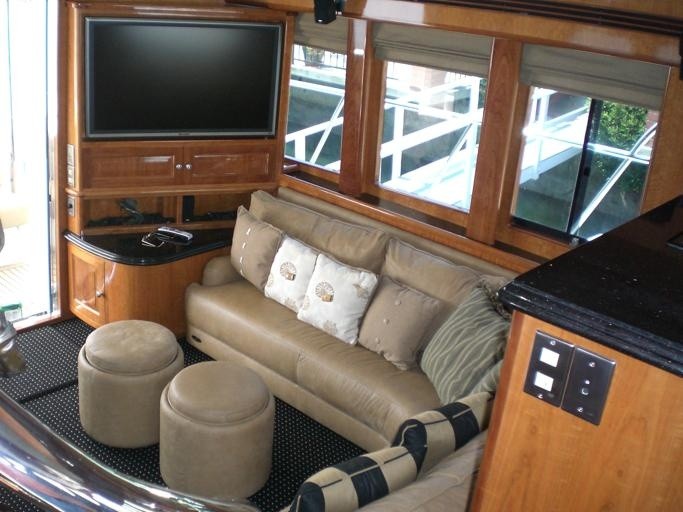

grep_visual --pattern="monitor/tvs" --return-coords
[84,16,284,140]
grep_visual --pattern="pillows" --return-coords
[288,445,428,512]
[414,286,510,406]
[263,232,321,314]
[356,271,442,372]
[296,252,381,347]
[229,205,286,294]
[390,391,495,482]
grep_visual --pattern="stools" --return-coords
[77,320,185,449]
[158,360,278,499]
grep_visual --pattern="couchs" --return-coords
[185,186,526,512]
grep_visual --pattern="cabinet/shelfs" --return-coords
[57,0,296,240]
[62,226,235,342]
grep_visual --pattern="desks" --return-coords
[465,194,683,512]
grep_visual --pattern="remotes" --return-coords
[158,226,194,243]
